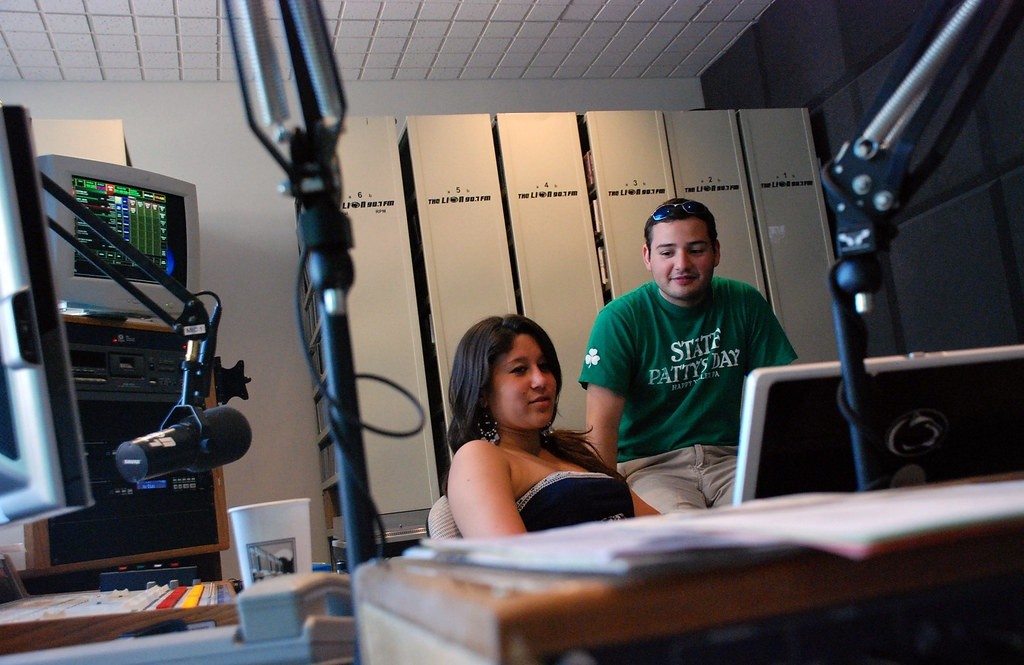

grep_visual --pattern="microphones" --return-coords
[233,0,351,316]
[115,407,251,485]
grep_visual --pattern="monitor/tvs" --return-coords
[0,101,93,530]
[36,154,203,324]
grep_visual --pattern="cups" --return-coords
[227,498,311,589]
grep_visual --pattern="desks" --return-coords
[349,470,1024,665]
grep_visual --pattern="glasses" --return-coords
[650,200,707,221]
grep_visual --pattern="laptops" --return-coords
[734,344,1024,505]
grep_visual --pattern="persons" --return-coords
[577,199,801,517]
[442,314,660,539]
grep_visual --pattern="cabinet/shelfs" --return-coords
[304,98,839,558]
[0,313,230,578]
